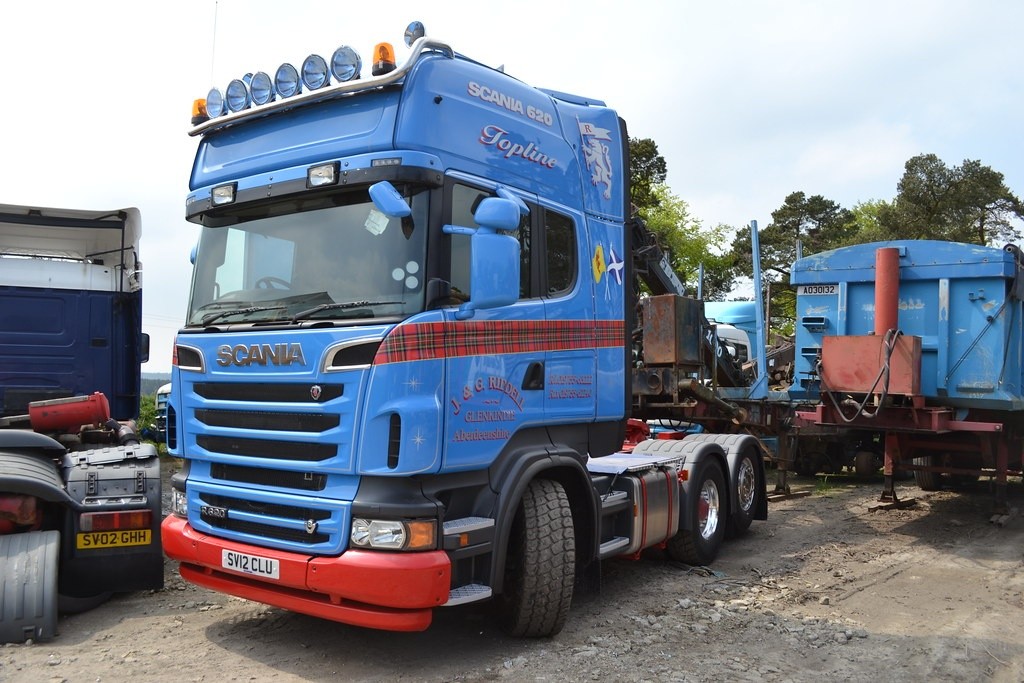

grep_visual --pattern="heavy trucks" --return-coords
[0,204,165,646]
[157,26,769,640]
